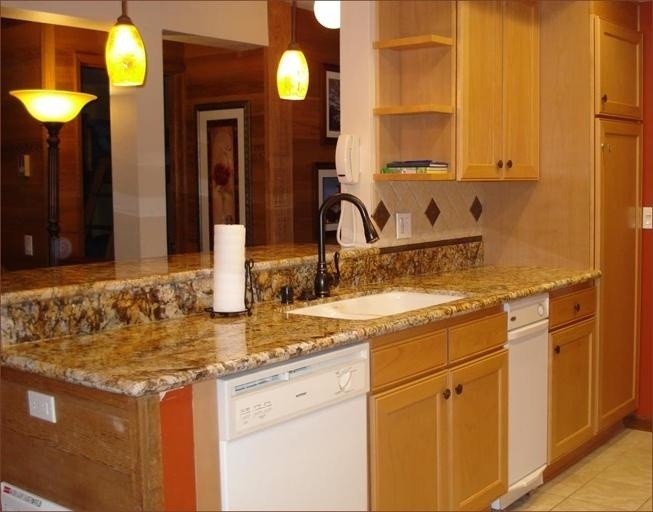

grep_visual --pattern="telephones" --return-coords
[335,135,360,184]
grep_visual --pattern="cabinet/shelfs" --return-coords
[376,1,542,182]
[370,284,600,512]
[504,1,642,441]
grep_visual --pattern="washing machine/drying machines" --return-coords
[218,343,371,512]
[488,291,549,511]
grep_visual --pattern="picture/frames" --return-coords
[194,99,248,252]
[324,62,342,140]
[313,161,341,235]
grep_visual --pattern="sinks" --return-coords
[278,284,475,319]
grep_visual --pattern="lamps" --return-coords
[313,0,341,30]
[105,1,145,87]
[9,90,98,266]
[275,0,309,101]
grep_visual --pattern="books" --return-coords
[380,159,447,173]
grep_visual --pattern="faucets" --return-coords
[315,192,380,297]
